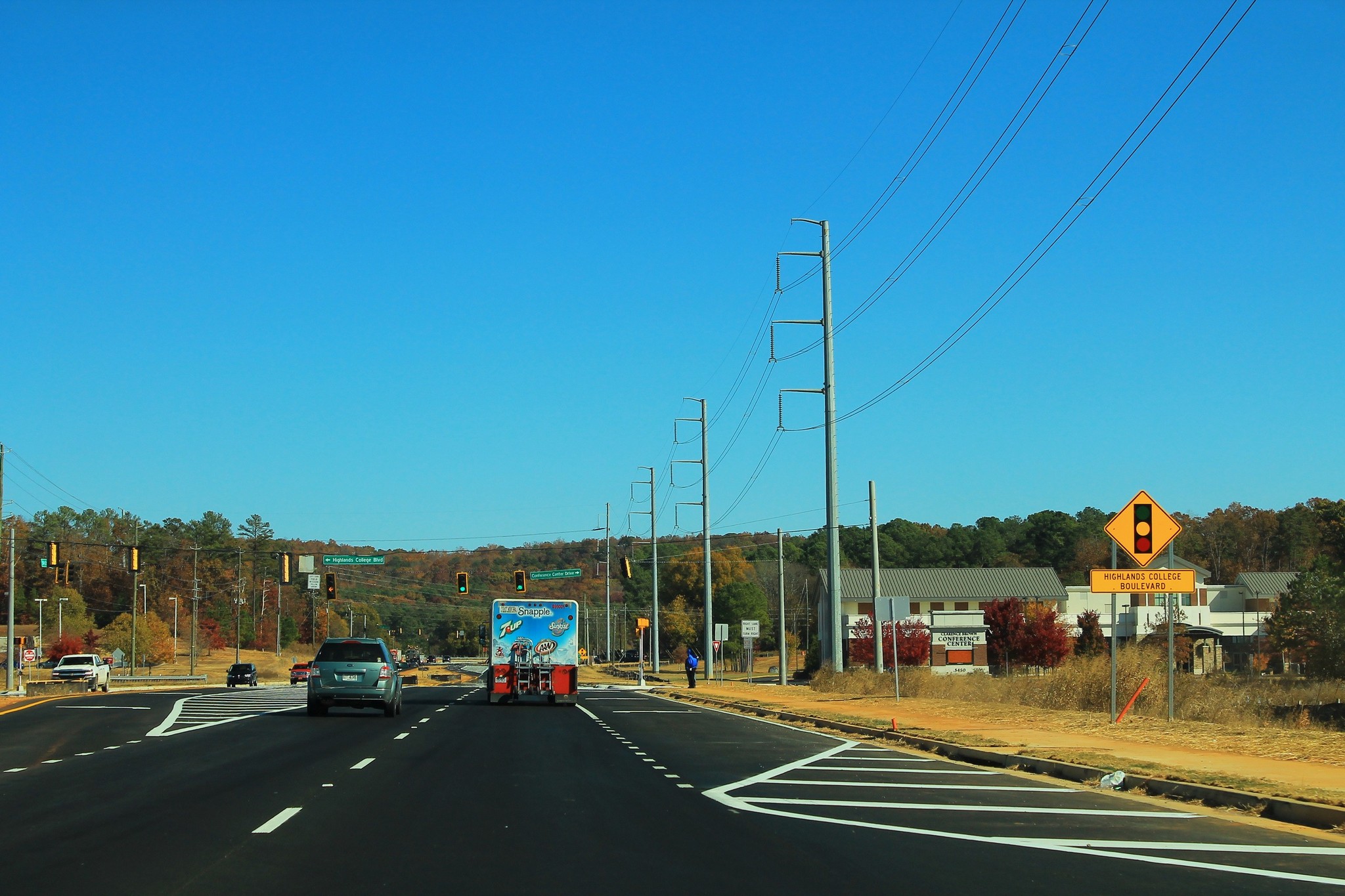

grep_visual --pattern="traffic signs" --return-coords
[1088,568,1196,595]
[529,567,582,580]
[323,554,385,565]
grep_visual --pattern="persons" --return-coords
[684,649,698,688]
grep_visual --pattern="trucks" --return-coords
[479,598,580,705]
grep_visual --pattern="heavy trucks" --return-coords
[405,646,420,662]
[389,648,401,661]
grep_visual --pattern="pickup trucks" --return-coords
[52,653,110,692]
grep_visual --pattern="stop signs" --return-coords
[23,650,36,661]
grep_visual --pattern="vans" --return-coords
[226,663,258,687]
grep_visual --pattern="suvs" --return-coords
[288,663,310,685]
[308,637,408,714]
[426,654,450,663]
[37,657,61,669]
[409,659,419,668]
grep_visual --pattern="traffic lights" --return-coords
[325,572,337,600]
[514,570,526,592]
[278,553,293,585]
[390,629,395,637]
[620,555,631,579]
[1134,503,1153,554]
[635,617,650,629]
[456,572,468,595]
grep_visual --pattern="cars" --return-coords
[0,660,24,669]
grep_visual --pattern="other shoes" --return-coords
[687,684,695,688]
